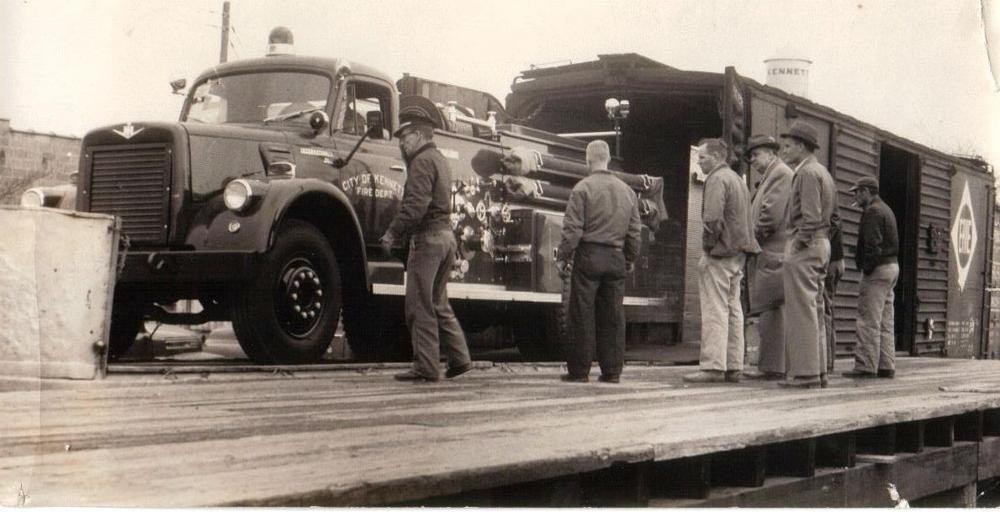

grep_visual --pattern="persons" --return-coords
[739,134,798,380]
[841,177,902,379]
[378,119,475,384]
[557,139,643,382]
[773,121,842,388]
[683,136,764,385]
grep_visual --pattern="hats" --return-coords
[780,119,821,149]
[848,177,880,192]
[743,135,780,155]
[394,113,437,137]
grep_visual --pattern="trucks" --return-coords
[65,42,679,378]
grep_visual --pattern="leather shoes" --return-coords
[777,376,821,388]
[842,370,878,377]
[822,377,830,387]
[743,371,776,377]
[395,371,438,381]
[879,370,894,377]
[445,364,472,378]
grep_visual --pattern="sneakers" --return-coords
[682,370,724,382]
[599,375,619,382]
[726,370,742,381]
[566,374,588,382]
